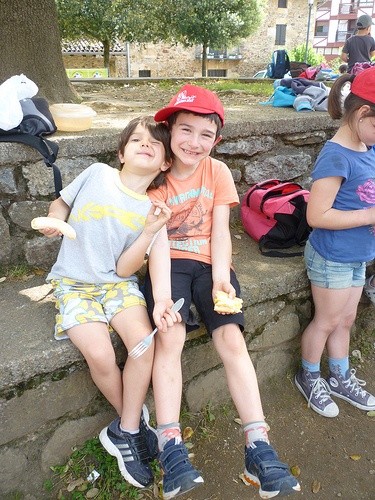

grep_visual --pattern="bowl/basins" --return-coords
[49,104,96,131]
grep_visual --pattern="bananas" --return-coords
[30,217,76,240]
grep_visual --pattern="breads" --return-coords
[213,291,243,315]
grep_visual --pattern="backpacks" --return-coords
[241,179,311,256]
[0,97,63,199]
[266,50,291,79]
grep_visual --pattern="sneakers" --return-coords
[139,403,160,461]
[294,367,339,417]
[324,367,375,412]
[97,417,154,489]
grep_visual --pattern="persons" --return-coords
[39,114,176,490]
[341,14,375,73]
[153,85,304,500]
[338,63,349,75]
[291,60,375,417]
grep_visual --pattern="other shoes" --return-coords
[245,440,302,500]
[158,438,204,500]
[363,275,375,306]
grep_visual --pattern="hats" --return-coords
[154,84,225,128]
[356,15,375,28]
[293,95,316,112]
[350,66,375,104]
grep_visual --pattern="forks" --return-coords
[128,298,185,359]
